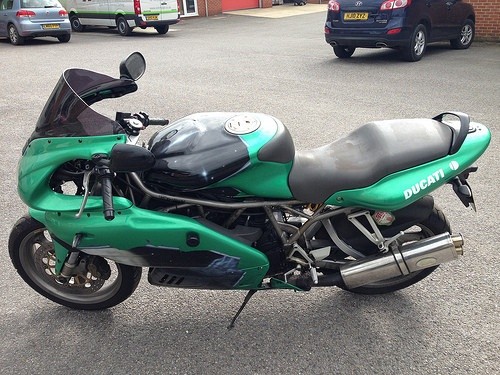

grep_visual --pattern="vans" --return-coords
[62,0,180,36]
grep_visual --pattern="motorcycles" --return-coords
[6,52,491,334]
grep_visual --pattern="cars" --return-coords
[325,0,476,61]
[1,0,72,45]
